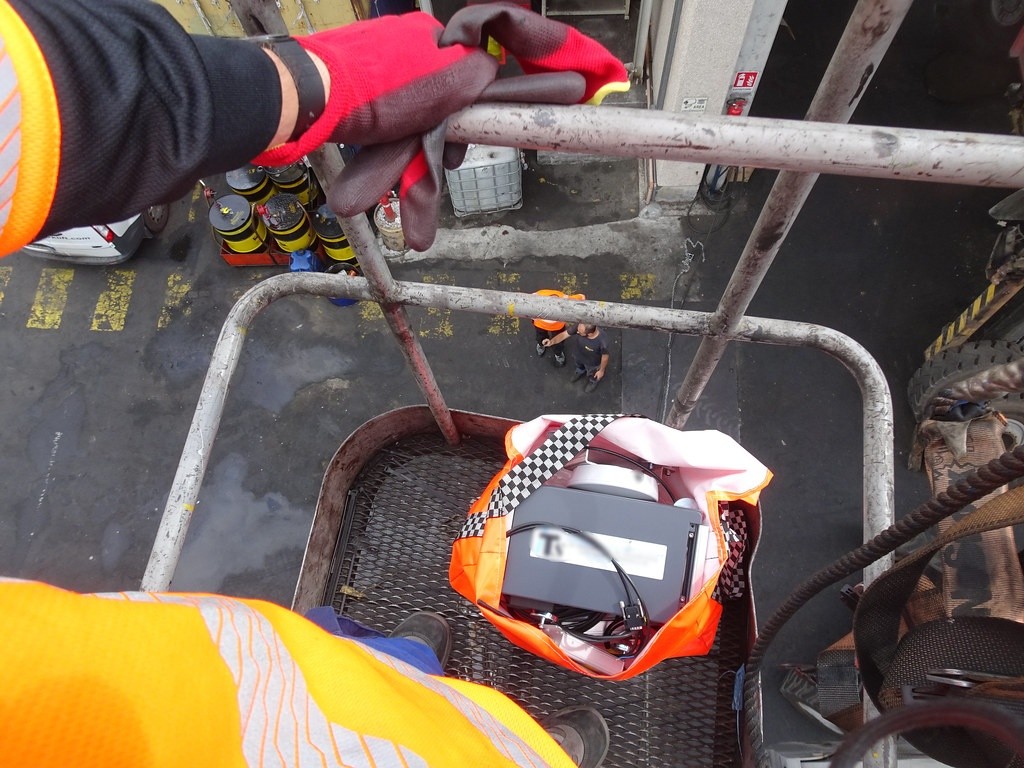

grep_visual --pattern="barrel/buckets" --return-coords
[208,162,357,262]
[375,199,411,251]
[321,264,362,307]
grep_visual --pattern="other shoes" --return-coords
[385,610,453,674]
[584,382,598,394]
[536,703,611,768]
[553,351,567,368]
[536,342,546,358]
[570,373,584,383]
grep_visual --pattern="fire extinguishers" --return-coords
[727,97,747,116]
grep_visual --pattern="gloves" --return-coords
[245,12,498,170]
[325,3,630,254]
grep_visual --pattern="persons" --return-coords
[513,290,585,367]
[0,0,610,768]
[542,323,611,392]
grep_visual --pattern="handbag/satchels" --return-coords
[448,415,775,683]
